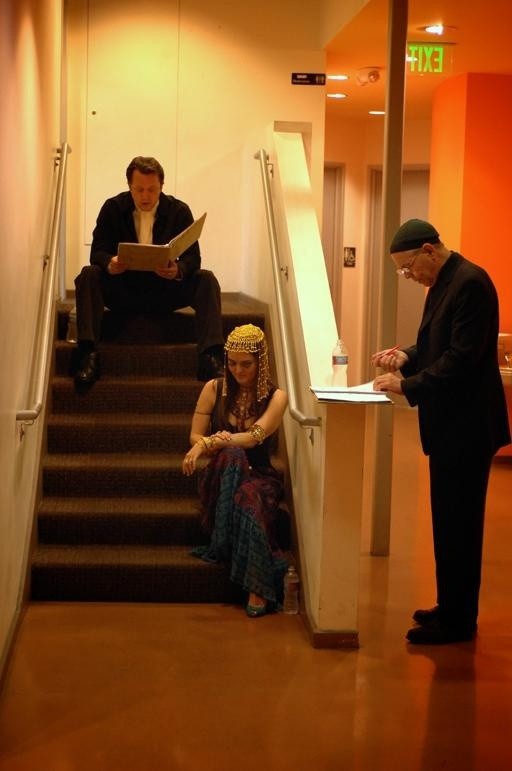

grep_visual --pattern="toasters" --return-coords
[331,337,348,389]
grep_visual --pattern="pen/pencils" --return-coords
[379,344,401,359]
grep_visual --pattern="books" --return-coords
[116,213,208,271]
[309,377,397,408]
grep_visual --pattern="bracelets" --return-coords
[246,424,264,446]
[203,432,215,449]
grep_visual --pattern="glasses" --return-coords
[396,252,418,275]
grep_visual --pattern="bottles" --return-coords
[65,305,77,344]
[280,565,300,615]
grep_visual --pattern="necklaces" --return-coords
[228,383,254,427]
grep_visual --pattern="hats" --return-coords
[390,219,440,253]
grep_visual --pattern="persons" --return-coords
[180,323,289,618]
[73,154,223,387]
[404,645,481,770]
[369,218,512,644]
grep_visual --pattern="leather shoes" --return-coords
[197,354,225,382]
[246,603,266,617]
[405,605,477,644]
[79,350,97,382]
